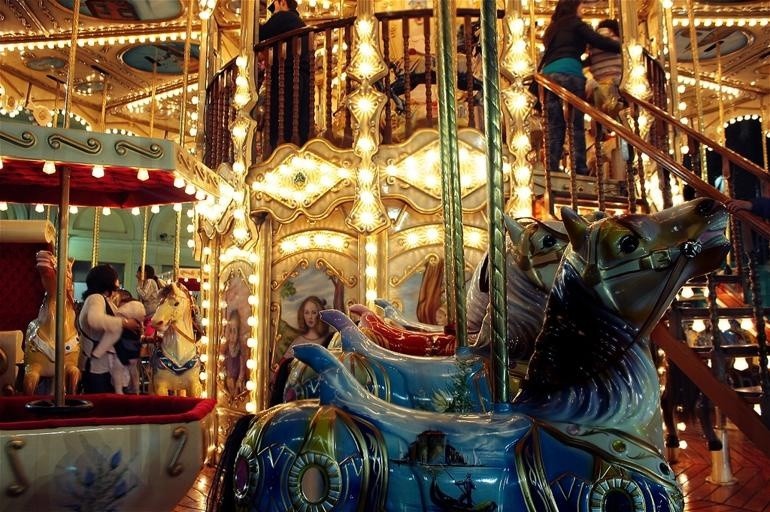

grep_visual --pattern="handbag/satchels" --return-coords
[528,80,539,111]
[114,329,142,366]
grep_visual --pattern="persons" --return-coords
[132,262,161,315]
[742,174,770,328]
[71,262,144,395]
[653,317,762,354]
[539,0,623,177]
[589,18,664,86]
[254,0,310,146]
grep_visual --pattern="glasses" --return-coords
[268,1,275,12]
